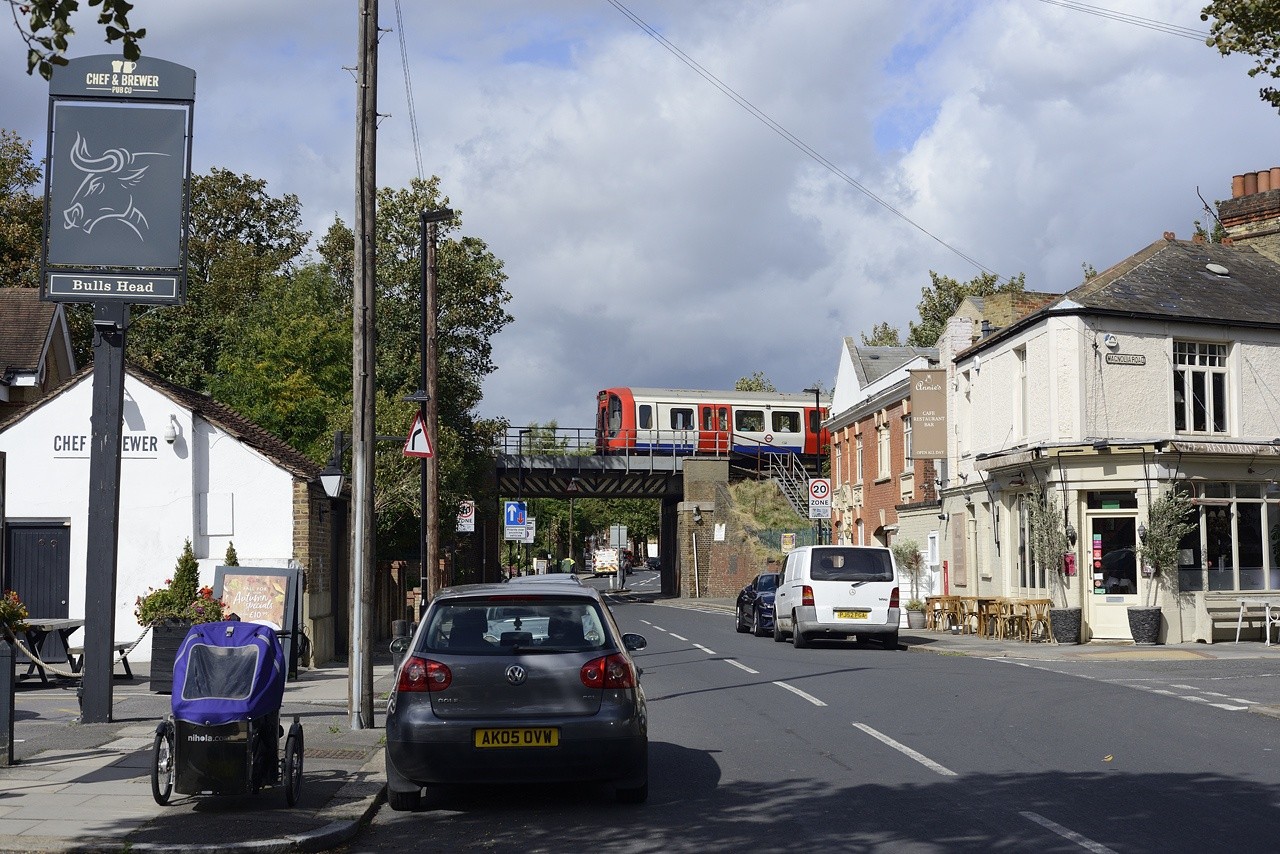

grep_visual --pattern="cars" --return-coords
[383,581,648,810]
[488,572,600,646]
[625,559,632,573]
[585,553,594,566]
[735,573,781,636]
[646,555,661,570]
[1101,549,1137,593]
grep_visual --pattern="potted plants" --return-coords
[905,600,926,629]
[1127,477,1199,643]
[1019,475,1081,643]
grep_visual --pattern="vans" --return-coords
[622,549,633,561]
[772,545,901,647]
[593,548,626,577]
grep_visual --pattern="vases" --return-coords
[150,616,240,691]
[0,639,15,766]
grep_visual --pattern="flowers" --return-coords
[0,588,28,637]
[134,536,232,627]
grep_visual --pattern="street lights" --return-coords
[321,208,455,614]
[518,430,533,577]
[803,388,822,546]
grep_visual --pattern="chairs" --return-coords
[820,558,833,568]
[450,610,502,648]
[926,596,1055,644]
[543,614,593,649]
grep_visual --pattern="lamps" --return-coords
[1137,522,1146,545]
[310,454,345,515]
[1092,441,1109,450]
[1066,521,1077,549]
[835,525,844,541]
[844,526,854,543]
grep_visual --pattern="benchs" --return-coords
[1193,589,1280,644]
[67,642,133,686]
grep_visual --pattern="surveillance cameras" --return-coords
[164,423,176,445]
[693,515,702,523]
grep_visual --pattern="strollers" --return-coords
[151,618,305,806]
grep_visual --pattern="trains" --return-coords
[594,387,831,460]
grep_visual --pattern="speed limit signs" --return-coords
[810,480,828,498]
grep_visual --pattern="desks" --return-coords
[928,597,967,632]
[16,618,85,689]
[1234,598,1280,647]
[988,600,1028,641]
[961,596,998,640]
